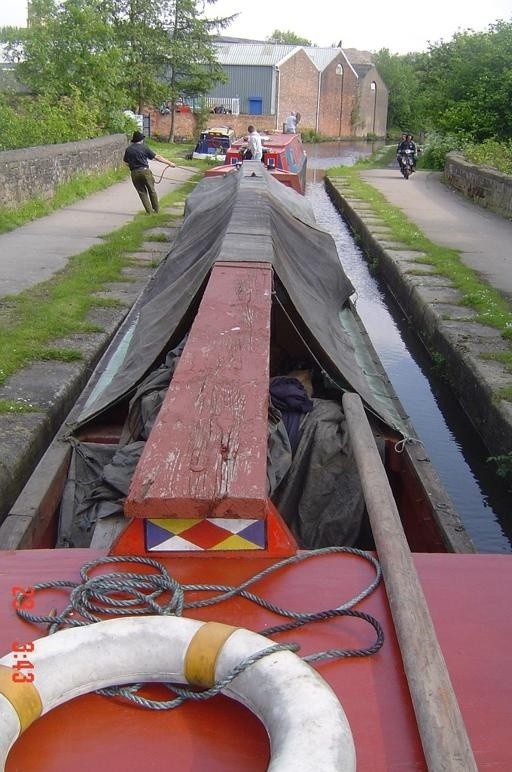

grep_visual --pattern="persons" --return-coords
[146,100,193,114]
[123,130,176,216]
[286,112,297,134]
[241,125,262,161]
[212,104,232,115]
[396,133,418,173]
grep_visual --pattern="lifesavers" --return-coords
[0,616,356,772]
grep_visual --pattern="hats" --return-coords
[131,131,145,142]
